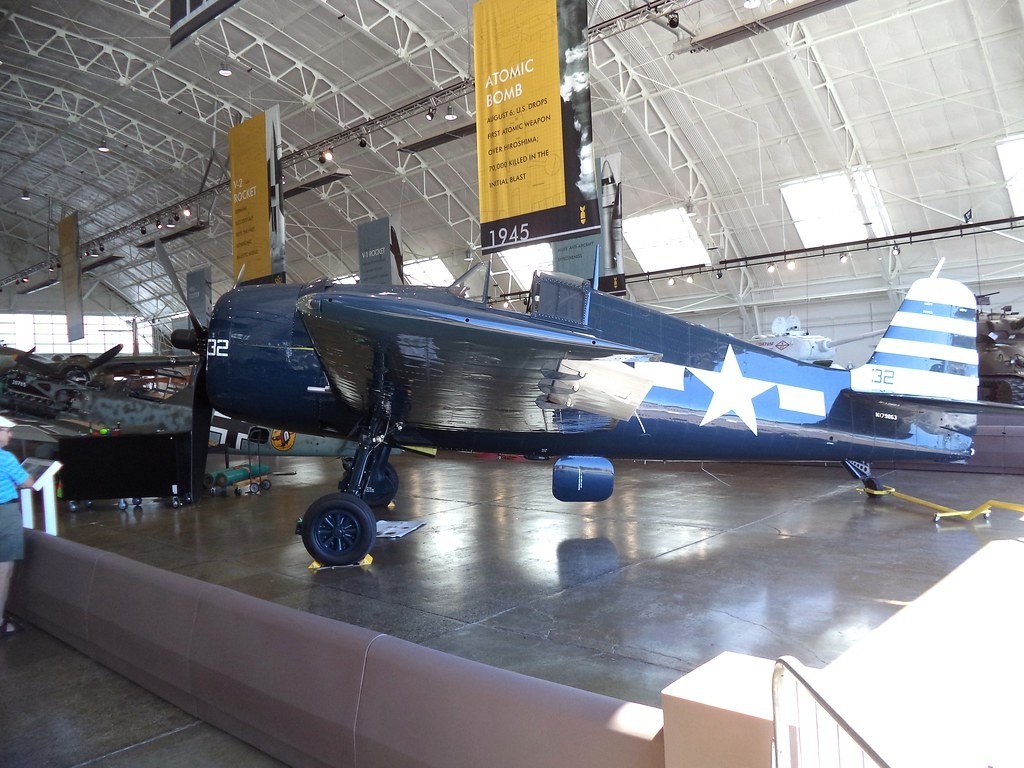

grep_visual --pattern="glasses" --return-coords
[0,428,12,432]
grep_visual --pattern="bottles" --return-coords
[57,480,64,498]
[87,422,93,436]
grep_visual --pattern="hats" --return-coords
[0,415,18,428]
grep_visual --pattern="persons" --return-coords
[0,416,35,634]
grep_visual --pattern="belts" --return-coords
[0,499,19,505]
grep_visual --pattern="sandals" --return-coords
[0,616,25,637]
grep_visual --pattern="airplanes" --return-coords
[0,380,405,493]
[154,232,981,567]
[0,343,176,415]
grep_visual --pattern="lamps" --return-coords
[444,98,458,121]
[316,146,336,164]
[686,273,695,284]
[766,261,777,273]
[667,276,676,286]
[839,252,849,264]
[13,203,201,288]
[891,244,901,257]
[787,259,797,271]
[95,135,111,153]
[502,301,510,308]
[356,134,369,149]
[218,57,233,78]
[715,269,723,279]
[19,190,33,202]
[425,106,438,122]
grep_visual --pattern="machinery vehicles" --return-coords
[748,316,888,371]
[974,291,1024,406]
